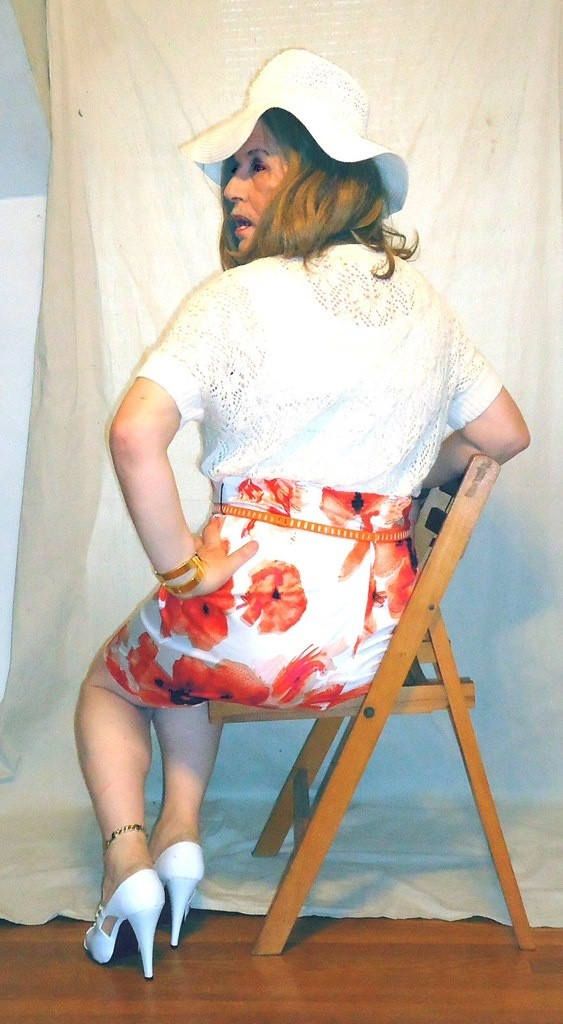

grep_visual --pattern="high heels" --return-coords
[153,841,205,948]
[85,869,165,982]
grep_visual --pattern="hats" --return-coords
[179,49,408,218]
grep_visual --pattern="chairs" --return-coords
[209,455,538,955]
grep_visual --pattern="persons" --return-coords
[68,46,532,978]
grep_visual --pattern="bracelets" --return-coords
[153,551,201,582]
[162,564,203,597]
